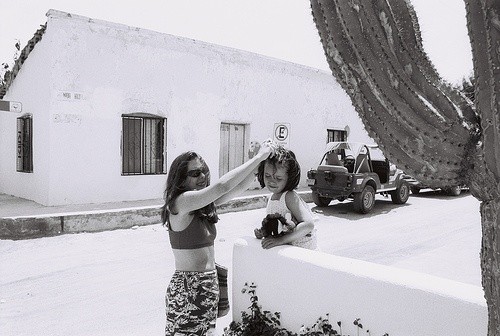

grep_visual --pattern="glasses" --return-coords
[186,168,209,177]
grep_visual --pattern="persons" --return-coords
[160,140,276,336]
[252,146,316,252]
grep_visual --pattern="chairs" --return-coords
[355,154,371,173]
[326,153,344,167]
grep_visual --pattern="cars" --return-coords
[401,172,470,197]
[307,143,411,214]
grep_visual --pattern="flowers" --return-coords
[260,212,297,240]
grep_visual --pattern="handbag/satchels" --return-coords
[215,262,230,318]
